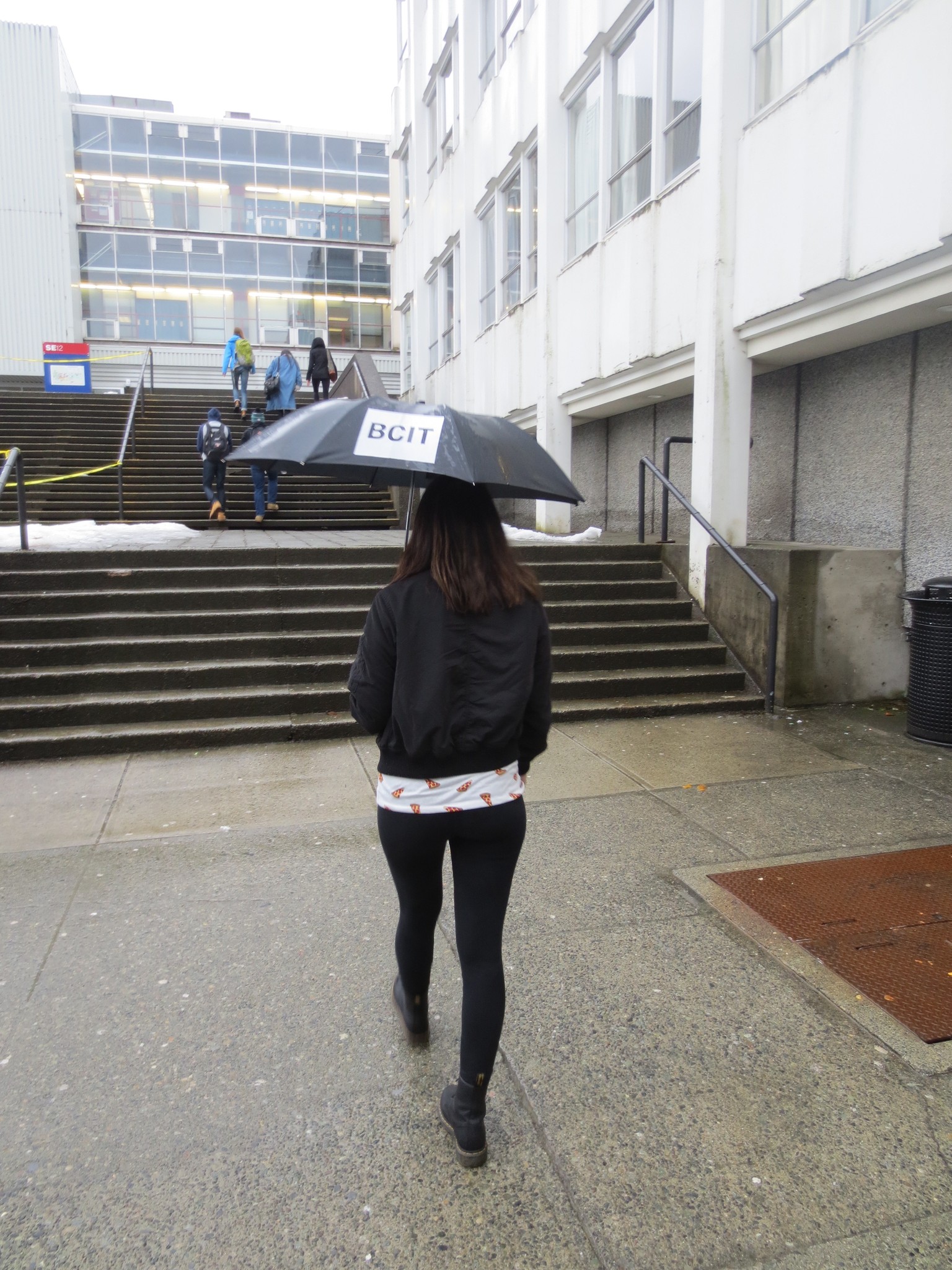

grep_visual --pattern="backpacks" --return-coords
[203,422,227,461]
[248,426,264,438]
[235,339,256,367]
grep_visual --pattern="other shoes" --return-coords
[218,512,226,522]
[210,501,221,519]
[267,503,279,510]
[233,405,239,413]
[240,415,247,419]
[255,515,263,522]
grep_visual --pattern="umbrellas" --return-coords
[217,390,587,561]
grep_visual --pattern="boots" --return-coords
[437,1072,490,1168]
[391,972,430,1044]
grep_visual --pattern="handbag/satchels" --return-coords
[327,348,336,382]
[264,376,280,394]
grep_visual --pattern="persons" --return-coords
[198,407,232,524]
[305,337,337,400]
[220,326,257,421]
[264,347,303,416]
[344,479,558,1167]
[239,406,280,522]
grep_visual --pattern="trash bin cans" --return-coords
[897,575,952,748]
